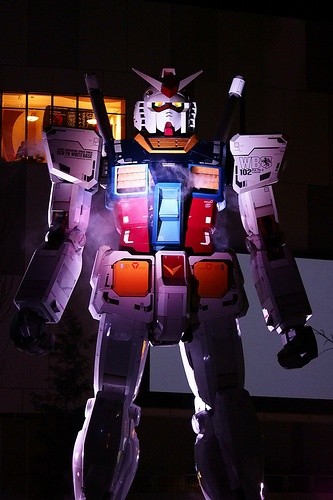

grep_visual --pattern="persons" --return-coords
[8,67,321,499]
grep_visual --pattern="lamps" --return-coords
[26,113,39,122]
[86,118,98,124]
